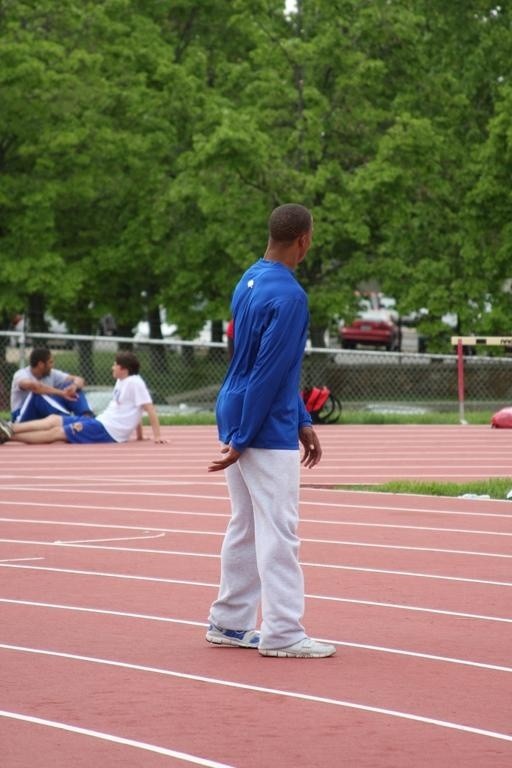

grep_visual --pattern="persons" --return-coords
[0,352,168,444]
[205,203,336,658]
[10,349,96,424]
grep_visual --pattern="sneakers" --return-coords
[205,624,263,649]
[258,637,336,659]
[0,418,16,444]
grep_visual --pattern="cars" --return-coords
[339,308,402,351]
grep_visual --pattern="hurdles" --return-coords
[451,336,512,425]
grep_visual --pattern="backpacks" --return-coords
[299,385,342,425]
